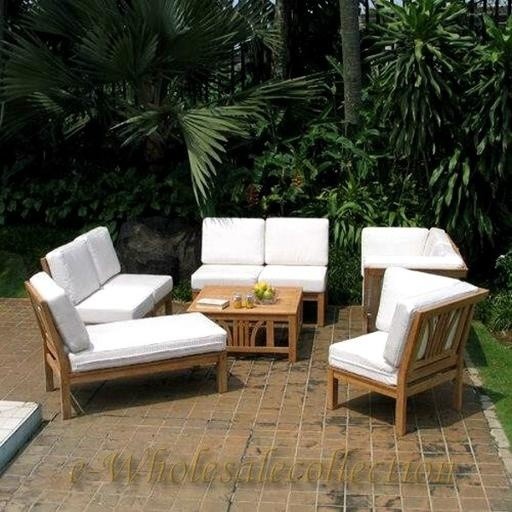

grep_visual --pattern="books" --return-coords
[195,297,229,310]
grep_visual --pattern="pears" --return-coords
[253,281,276,299]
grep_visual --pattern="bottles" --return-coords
[246,296,254,309]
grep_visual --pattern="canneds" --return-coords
[245,294,253,308]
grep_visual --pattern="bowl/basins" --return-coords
[255,296,279,305]
